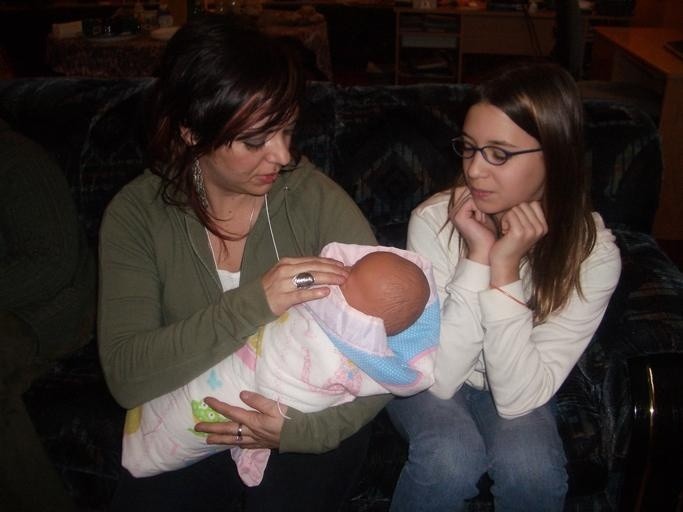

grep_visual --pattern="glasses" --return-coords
[450,136,542,167]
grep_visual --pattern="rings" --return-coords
[234,423,244,443]
[291,272,315,289]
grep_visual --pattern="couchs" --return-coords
[0,74,683,512]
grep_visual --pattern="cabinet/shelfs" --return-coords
[393,7,683,241]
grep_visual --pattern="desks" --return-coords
[48,8,330,74]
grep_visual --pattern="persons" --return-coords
[95,21,394,512]
[337,248,430,339]
[0,129,99,509]
[381,52,623,511]
